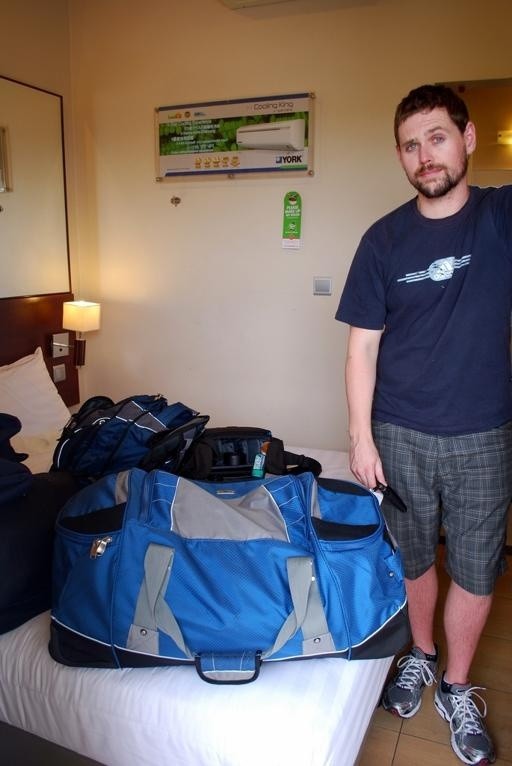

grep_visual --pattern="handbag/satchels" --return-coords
[48,468,412,685]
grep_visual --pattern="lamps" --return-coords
[61,297,102,370]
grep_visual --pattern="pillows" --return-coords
[0,345,74,476]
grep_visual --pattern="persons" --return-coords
[332,81,511,764]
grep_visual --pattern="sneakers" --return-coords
[381,642,441,718]
[433,671,496,766]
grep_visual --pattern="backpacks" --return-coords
[48,396,322,483]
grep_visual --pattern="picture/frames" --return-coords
[1,74,72,300]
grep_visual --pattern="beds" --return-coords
[1,293,406,765]
